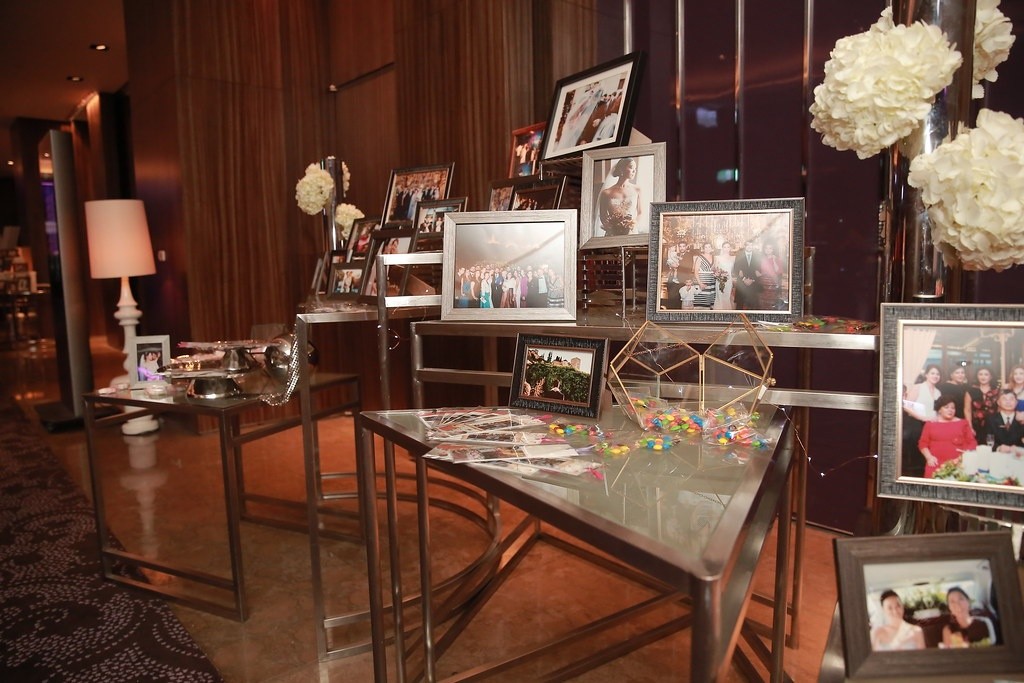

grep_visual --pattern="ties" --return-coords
[747,254,750,267]
[1007,417,1010,430]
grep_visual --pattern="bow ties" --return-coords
[539,277,543,279]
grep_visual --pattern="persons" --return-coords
[396,185,438,220]
[596,158,650,235]
[342,271,355,292]
[942,588,997,648]
[390,239,398,254]
[492,188,543,210]
[516,139,540,172]
[667,240,782,310]
[562,82,622,146]
[904,364,1024,480]
[142,351,161,379]
[420,214,444,233]
[455,264,564,308]
[871,591,926,651]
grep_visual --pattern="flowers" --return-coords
[293,154,365,241]
[808,1,1024,272]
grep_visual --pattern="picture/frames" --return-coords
[508,330,610,420]
[876,302,1024,509]
[311,50,805,324]
[127,335,171,390]
[833,529,1024,683]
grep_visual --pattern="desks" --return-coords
[407,304,879,683]
[360,400,796,683]
[296,251,503,683]
[83,373,370,622]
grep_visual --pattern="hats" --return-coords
[425,214,434,218]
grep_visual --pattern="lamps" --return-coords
[82,199,159,436]
